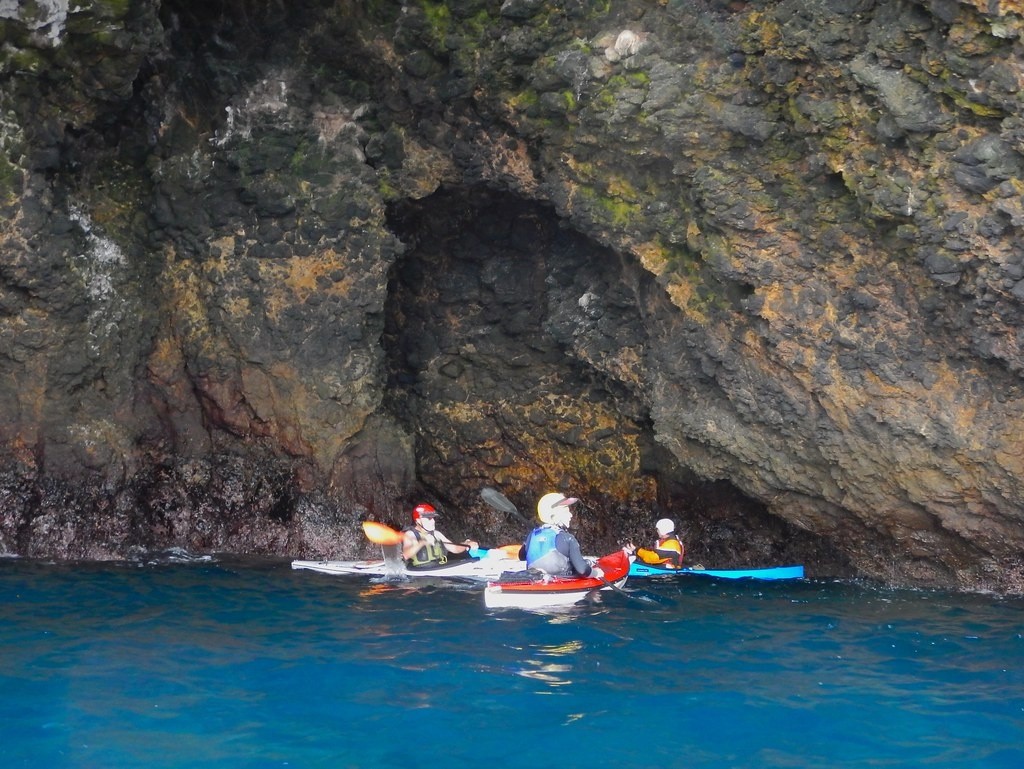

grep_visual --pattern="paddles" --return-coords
[361,520,522,560]
[480,485,630,597]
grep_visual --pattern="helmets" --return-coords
[538,493,578,524]
[413,504,440,523]
[656,519,675,536]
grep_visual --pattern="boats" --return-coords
[291,547,508,577]
[628,561,806,585]
[485,550,630,608]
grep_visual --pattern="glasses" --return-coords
[426,518,436,521]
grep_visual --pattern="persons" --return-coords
[518,492,592,576]
[402,504,479,567]
[627,518,684,569]
